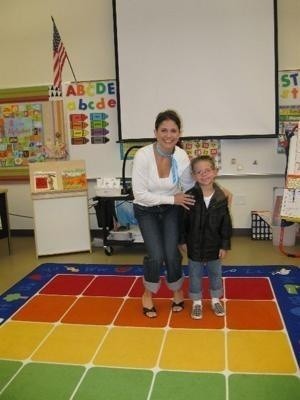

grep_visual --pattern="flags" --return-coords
[52,25,67,90]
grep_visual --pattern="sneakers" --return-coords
[190,304,203,320]
[211,302,226,317]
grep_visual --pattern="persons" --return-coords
[132,110,232,319]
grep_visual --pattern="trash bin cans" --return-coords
[271,222,299,247]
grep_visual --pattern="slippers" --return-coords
[143,305,158,318]
[172,300,185,313]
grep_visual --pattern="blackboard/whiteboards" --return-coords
[59,68,300,181]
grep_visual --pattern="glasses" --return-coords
[193,168,214,175]
